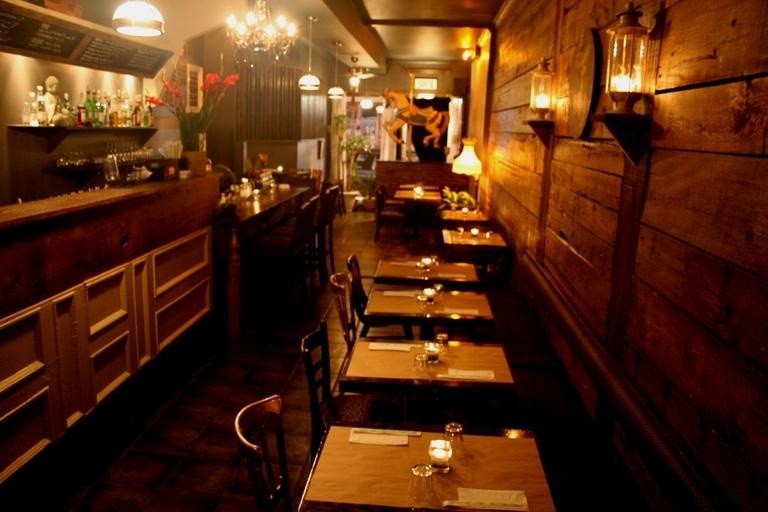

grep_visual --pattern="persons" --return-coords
[43,75,61,124]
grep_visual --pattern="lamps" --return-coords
[110,0,166,40]
[524,54,560,156]
[326,38,347,100]
[348,55,385,117]
[222,0,303,73]
[598,0,667,168]
[297,15,321,91]
[450,136,484,205]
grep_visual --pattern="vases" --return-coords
[180,129,209,180]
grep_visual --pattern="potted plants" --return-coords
[361,177,376,212]
[340,135,372,213]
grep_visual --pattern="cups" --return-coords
[450,205,480,217]
[427,439,453,474]
[414,183,423,194]
[408,465,437,501]
[443,423,466,459]
[456,226,492,238]
[416,254,437,274]
[179,169,191,179]
[239,182,252,198]
[413,332,450,370]
[416,284,446,310]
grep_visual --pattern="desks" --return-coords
[330,253,514,418]
[375,183,508,262]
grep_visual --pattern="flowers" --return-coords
[138,49,241,154]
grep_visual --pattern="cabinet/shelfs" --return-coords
[7,122,159,177]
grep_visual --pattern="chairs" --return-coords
[296,320,398,473]
[230,393,292,511]
[270,184,337,323]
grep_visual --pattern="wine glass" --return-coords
[54,139,154,186]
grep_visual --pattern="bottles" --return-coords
[24,84,153,127]
